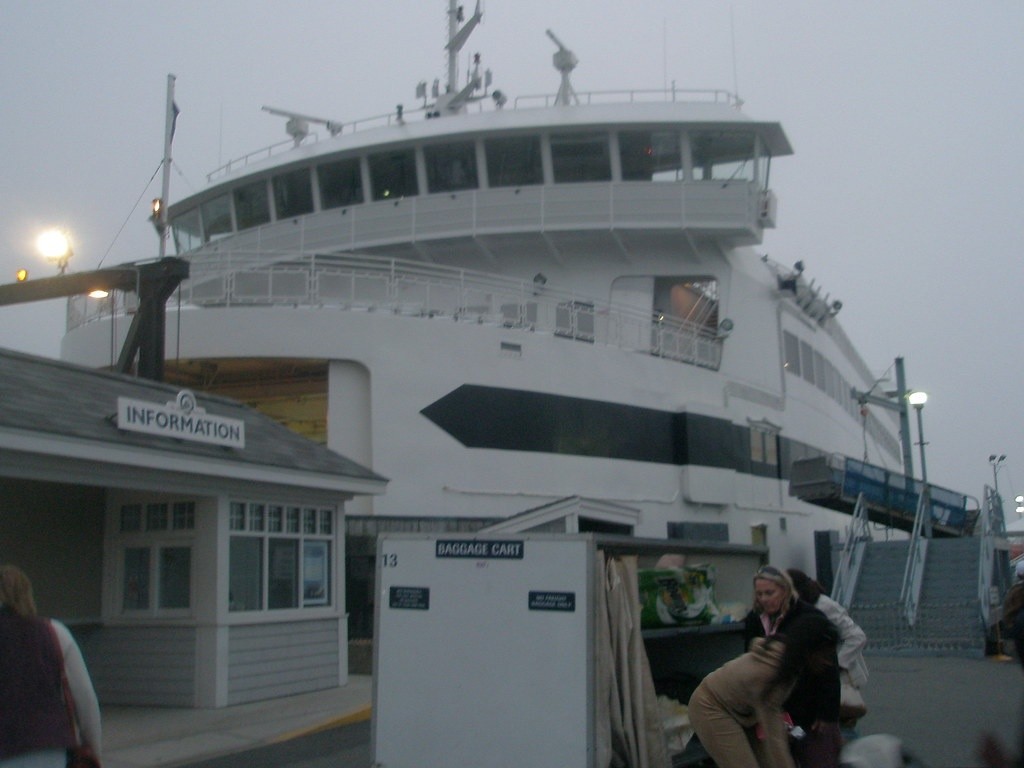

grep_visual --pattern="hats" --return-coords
[1016,560,1024,576]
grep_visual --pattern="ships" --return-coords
[59,0,917,623]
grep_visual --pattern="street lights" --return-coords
[908,391,933,537]
[986,451,1007,534]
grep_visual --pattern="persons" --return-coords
[742,563,842,768]
[0,563,102,768]
[786,569,868,730]
[1000,560,1024,663]
[687,613,843,768]
